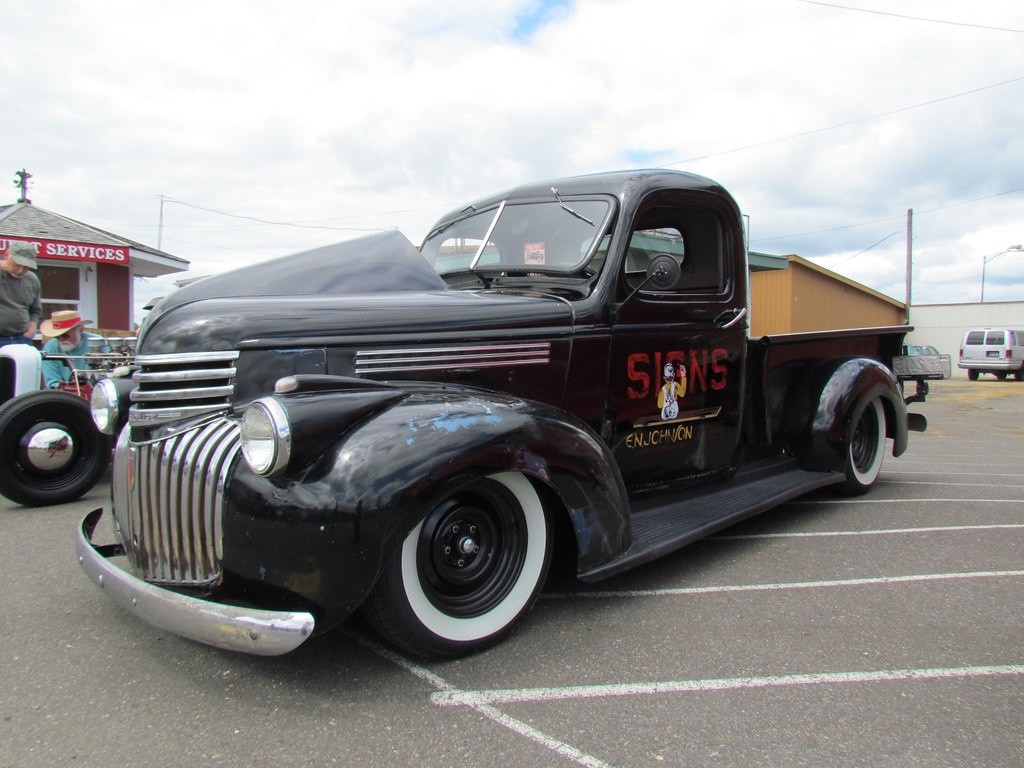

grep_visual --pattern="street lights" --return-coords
[981,245,1024,302]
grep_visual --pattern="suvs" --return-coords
[957,328,1024,381]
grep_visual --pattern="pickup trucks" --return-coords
[69,165,948,658]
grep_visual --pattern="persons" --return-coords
[40,311,112,389]
[0,240,41,348]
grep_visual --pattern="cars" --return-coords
[0,344,142,506]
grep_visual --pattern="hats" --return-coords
[9,241,37,269]
[40,311,93,337]
